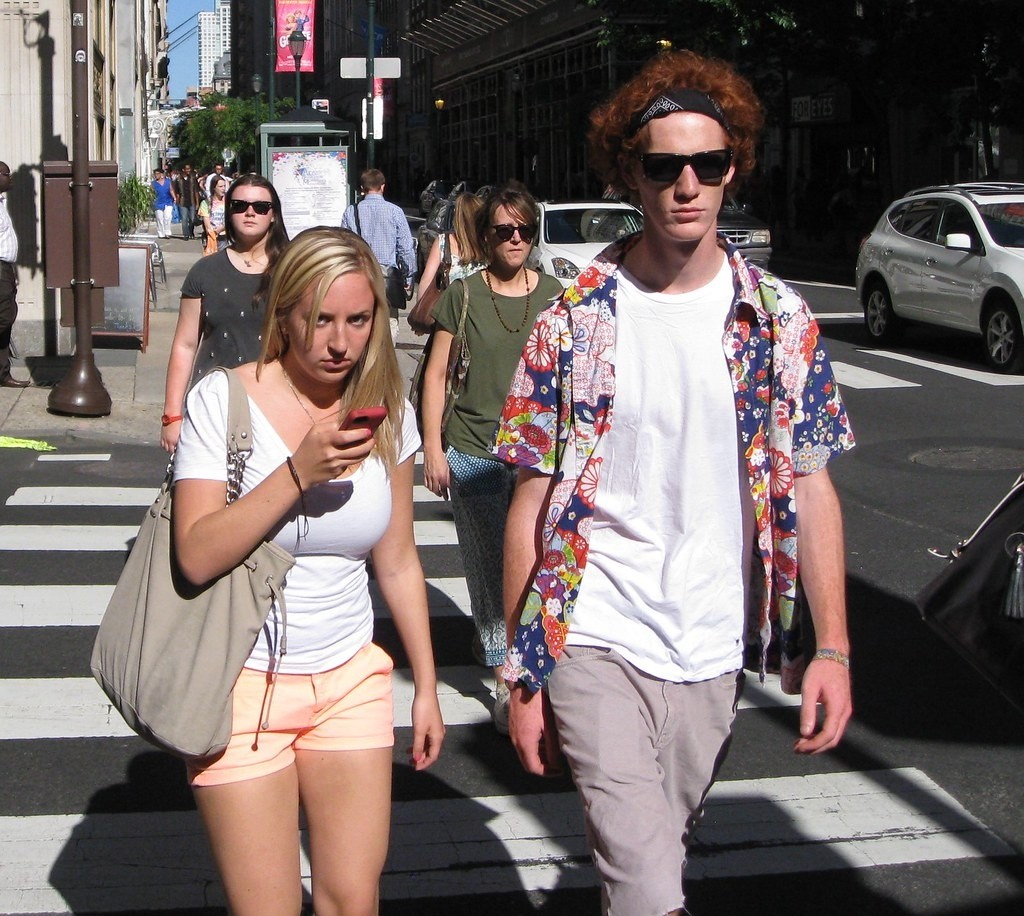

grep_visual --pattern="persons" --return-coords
[0,160,30,388]
[149,162,240,257]
[422,180,566,738]
[339,168,416,349]
[160,175,292,454]
[417,192,492,303]
[174,225,444,916]
[491,49,855,916]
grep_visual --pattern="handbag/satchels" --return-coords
[409,278,470,443]
[90,366,293,759]
[407,233,452,335]
[202,232,207,246]
[384,266,406,310]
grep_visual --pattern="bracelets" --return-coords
[286,456,310,541]
[174,199,177,201]
[812,648,850,669]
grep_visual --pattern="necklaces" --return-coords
[487,264,531,333]
[235,250,265,267]
[282,357,352,425]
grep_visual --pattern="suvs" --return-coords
[855,182,1024,375]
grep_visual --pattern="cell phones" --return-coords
[338,406,388,446]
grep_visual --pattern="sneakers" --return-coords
[492,683,509,733]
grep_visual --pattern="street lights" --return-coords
[250,73,265,177]
[288,30,307,150]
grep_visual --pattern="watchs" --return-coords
[161,414,183,426]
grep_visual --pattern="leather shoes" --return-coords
[0,379,31,387]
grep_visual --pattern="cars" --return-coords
[528,200,645,288]
[715,186,783,271]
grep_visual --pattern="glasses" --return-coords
[632,146,734,182]
[0,173,11,178]
[489,224,538,240]
[229,199,273,214]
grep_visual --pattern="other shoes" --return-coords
[158,235,170,239]
[189,228,194,238]
[185,235,189,241]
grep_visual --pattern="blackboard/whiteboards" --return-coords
[90,245,151,337]
